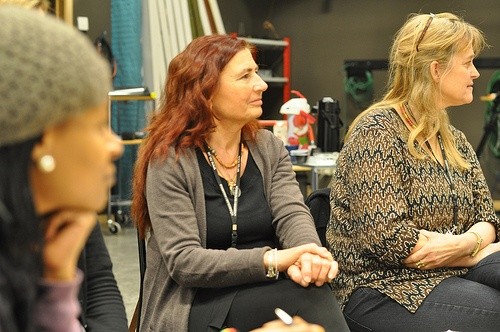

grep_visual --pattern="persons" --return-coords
[137,33,350,332]
[0,4,129,332]
[325,13,500,332]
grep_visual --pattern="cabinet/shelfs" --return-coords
[238,37,290,127]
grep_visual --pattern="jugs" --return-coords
[318,95,342,151]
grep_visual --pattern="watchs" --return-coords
[469,231,483,257]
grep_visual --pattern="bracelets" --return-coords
[266,247,280,279]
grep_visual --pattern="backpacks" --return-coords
[305,188,331,249]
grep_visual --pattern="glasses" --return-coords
[416,12,438,53]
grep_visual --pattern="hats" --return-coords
[0,5,110,146]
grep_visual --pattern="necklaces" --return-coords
[206,143,244,197]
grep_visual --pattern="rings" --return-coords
[416,260,424,268]
